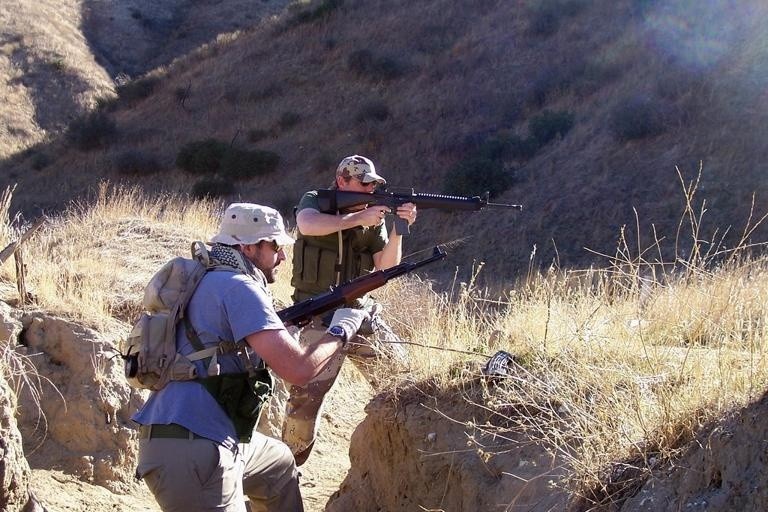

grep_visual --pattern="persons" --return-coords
[127,202,372,511]
[280,155,419,468]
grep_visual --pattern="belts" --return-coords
[138,424,210,440]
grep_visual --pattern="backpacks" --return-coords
[122,239,245,393]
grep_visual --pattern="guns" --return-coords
[276,237,475,328]
[317,188,523,235]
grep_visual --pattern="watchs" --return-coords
[323,326,346,338]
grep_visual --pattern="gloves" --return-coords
[325,307,372,346]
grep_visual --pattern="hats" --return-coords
[204,201,296,246]
[335,155,387,184]
